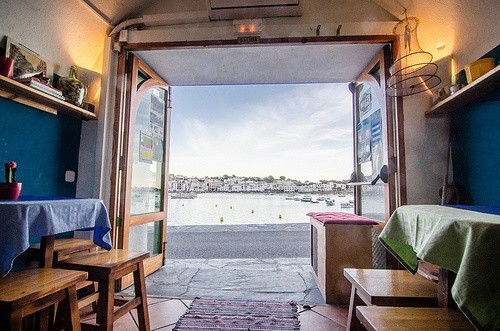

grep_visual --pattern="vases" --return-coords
[0,182,22,200]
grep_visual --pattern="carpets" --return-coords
[171,297,301,331]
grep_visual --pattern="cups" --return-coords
[449,84,460,95]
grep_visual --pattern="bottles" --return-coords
[59,65,87,107]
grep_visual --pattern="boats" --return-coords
[339,200,354,208]
[338,195,345,197]
[152,191,161,207]
[300,195,335,206]
[134,192,143,202]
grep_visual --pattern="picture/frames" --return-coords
[6,37,53,86]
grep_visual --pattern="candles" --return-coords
[451,57,456,84]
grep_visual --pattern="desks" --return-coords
[0,198,117,331]
[379,204,500,331]
[305,211,384,306]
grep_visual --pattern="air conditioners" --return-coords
[206,0,302,19]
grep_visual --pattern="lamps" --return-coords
[386,9,441,99]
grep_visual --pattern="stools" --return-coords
[408,331,408,331]
[0,236,150,331]
[344,261,473,331]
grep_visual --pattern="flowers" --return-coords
[6,162,19,182]
